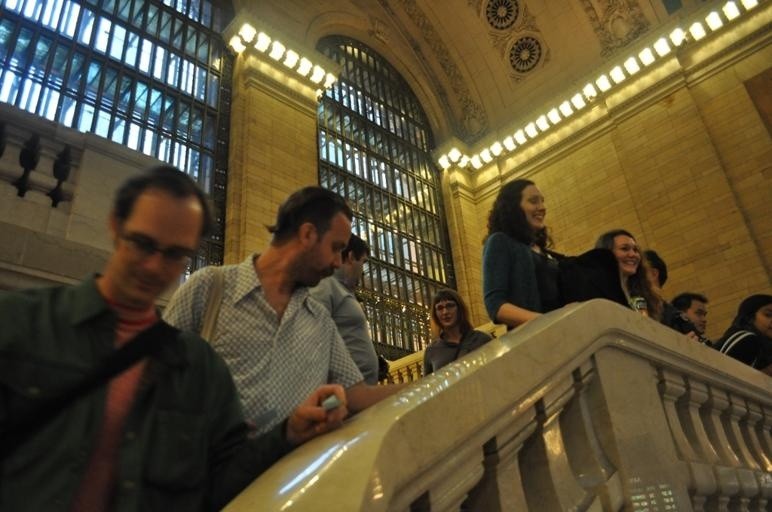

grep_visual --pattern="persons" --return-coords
[422,287,492,377]
[710,293,772,376]
[158,185,417,440]
[306,233,381,386]
[0,159,349,512]
[480,176,582,333]
[594,229,664,324]
[640,249,714,352]
[668,292,709,336]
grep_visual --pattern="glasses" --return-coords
[120,230,194,263]
